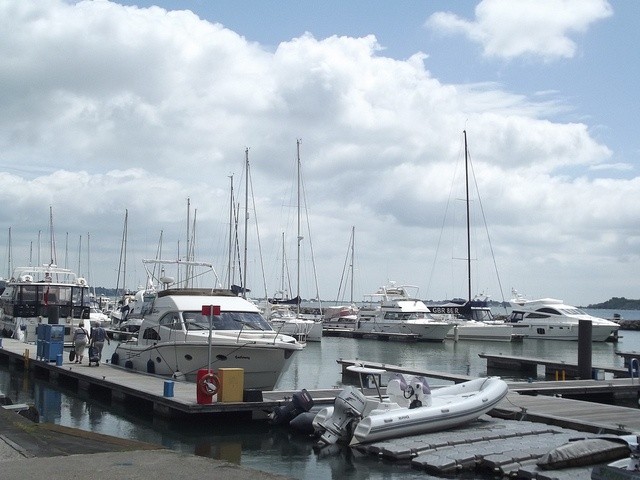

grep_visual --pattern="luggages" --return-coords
[88,342,100,367]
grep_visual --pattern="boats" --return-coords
[289,361,509,460]
[106,278,305,391]
[452,287,621,338]
[0,268,91,347]
[322,281,464,342]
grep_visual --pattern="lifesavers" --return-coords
[199,373,220,397]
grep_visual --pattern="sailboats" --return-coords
[64,208,130,326]
[321,226,357,328]
[216,138,322,342]
[427,131,508,324]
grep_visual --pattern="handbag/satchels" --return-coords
[69,351,75,361]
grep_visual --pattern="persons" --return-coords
[72,322,91,364]
[89,321,110,366]
